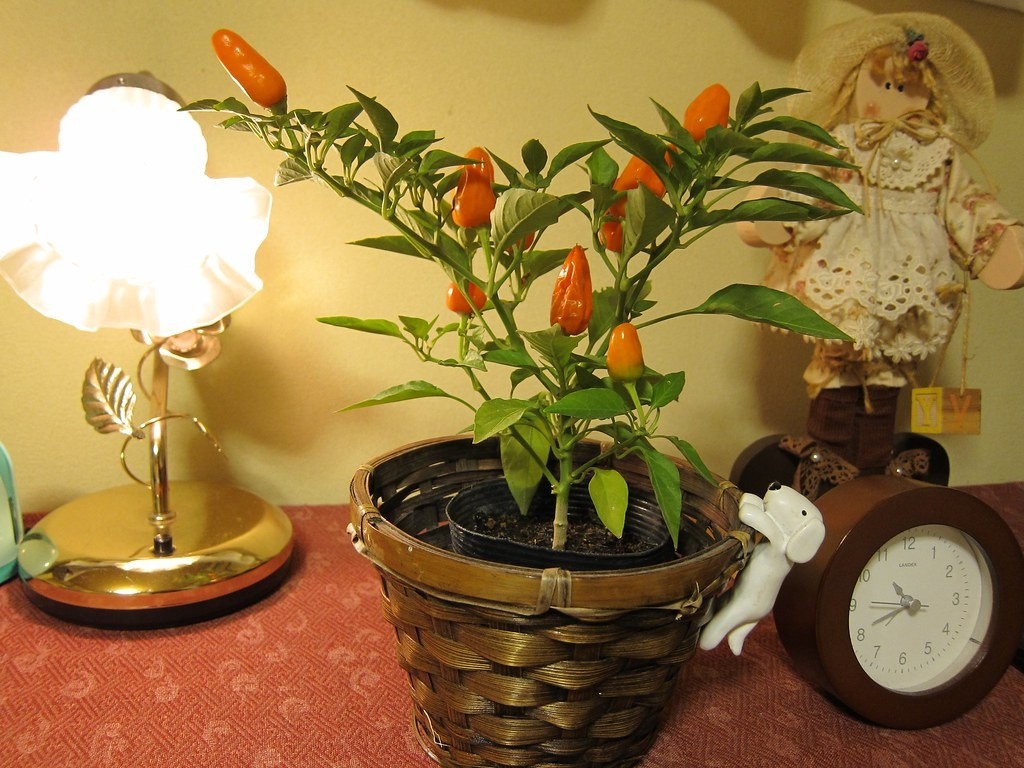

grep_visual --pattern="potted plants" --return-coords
[174,27,867,569]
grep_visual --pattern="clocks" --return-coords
[771,468,1024,731]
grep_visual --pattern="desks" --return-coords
[0,480,1024,768]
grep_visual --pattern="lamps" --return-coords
[0,68,298,632]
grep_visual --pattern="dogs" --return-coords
[699,481,826,655]
